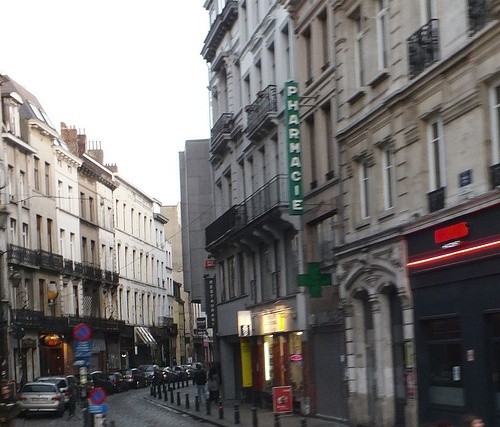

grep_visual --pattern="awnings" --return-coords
[135,327,156,345]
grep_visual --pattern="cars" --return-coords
[15,383,65,418]
[74,365,193,398]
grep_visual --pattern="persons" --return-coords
[208,367,220,402]
[193,365,207,406]
[461,414,486,427]
[172,358,177,366]
[67,378,78,417]
[1,370,8,380]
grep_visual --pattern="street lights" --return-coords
[104,305,117,371]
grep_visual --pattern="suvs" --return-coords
[33,377,80,409]
